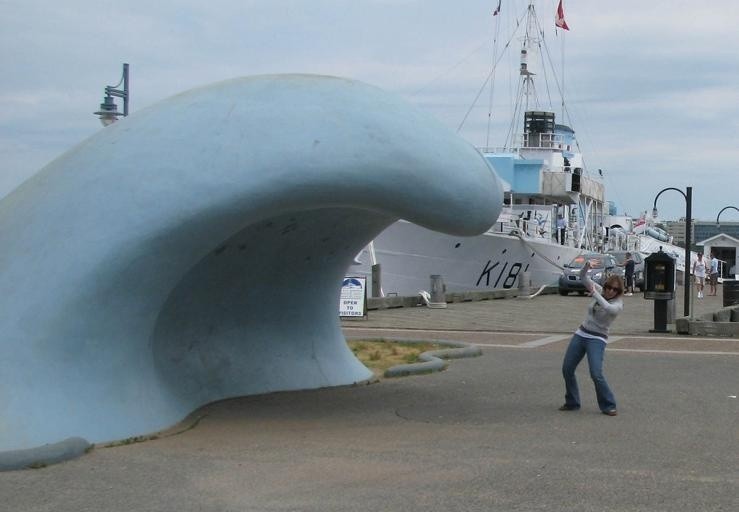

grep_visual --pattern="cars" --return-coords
[559,253,624,296]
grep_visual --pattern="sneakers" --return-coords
[697,292,703,298]
[624,292,632,296]
[608,410,617,416]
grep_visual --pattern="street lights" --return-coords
[93,61,129,129]
[652,186,693,318]
[716,206,738,228]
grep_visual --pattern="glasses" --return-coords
[606,283,618,293]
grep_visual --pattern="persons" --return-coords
[599,222,607,252]
[559,259,624,416]
[707,252,719,297]
[692,251,708,299]
[556,212,567,245]
[617,252,635,296]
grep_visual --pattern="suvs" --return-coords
[607,250,647,292]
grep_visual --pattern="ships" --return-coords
[349,1,697,313]
